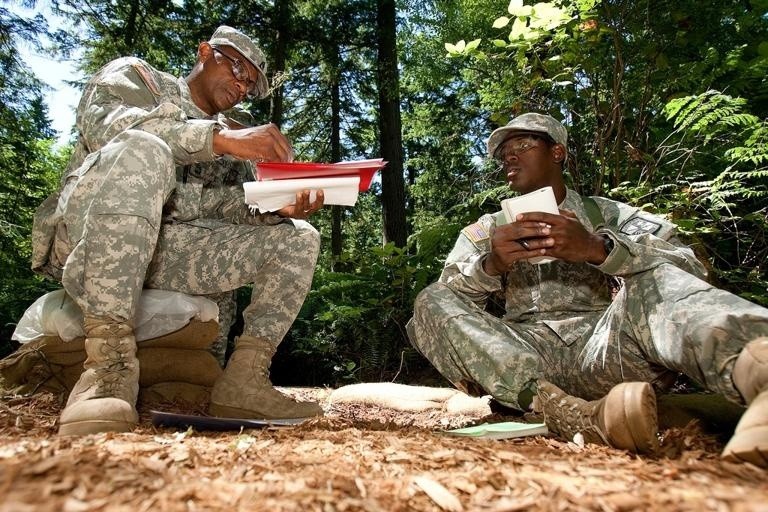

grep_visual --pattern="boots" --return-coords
[55,307,142,435]
[206,331,325,420]
[719,334,768,467]
[521,372,660,454]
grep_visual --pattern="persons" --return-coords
[26,21,327,435]
[405,111,767,468]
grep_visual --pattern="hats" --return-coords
[206,23,271,101]
[485,113,570,167]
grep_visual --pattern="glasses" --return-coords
[209,46,262,101]
[492,133,544,164]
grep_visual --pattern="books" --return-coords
[500,185,561,266]
[229,155,388,217]
[439,415,558,447]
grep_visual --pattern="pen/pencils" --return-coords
[519,239,530,249]
